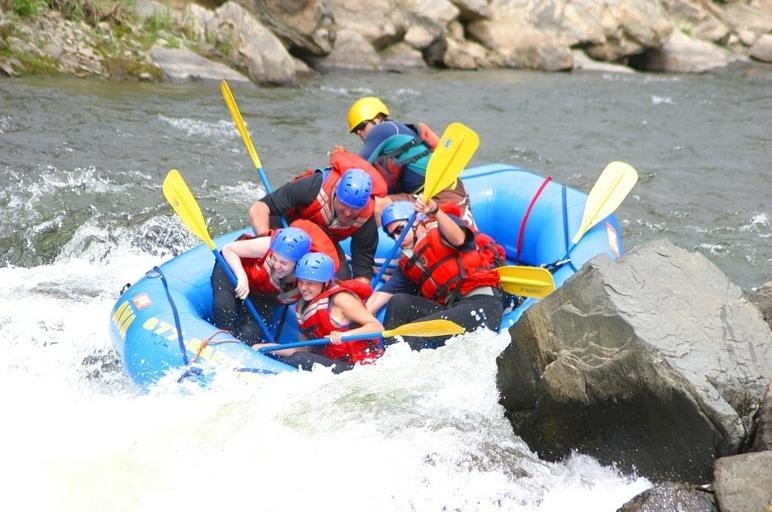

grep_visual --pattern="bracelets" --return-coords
[429,202,440,216]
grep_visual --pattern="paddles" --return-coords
[491,265,555,299]
[161,168,277,342]
[559,161,639,262]
[218,79,289,228]
[257,319,465,354]
[370,122,481,290]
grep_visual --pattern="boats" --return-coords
[110,162,622,410]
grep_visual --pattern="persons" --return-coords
[251,250,386,374]
[210,226,309,345]
[248,166,379,288]
[363,191,503,352]
[348,96,478,237]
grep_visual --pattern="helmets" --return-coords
[346,95,391,135]
[380,200,417,233]
[293,251,337,285]
[269,226,311,262]
[335,167,373,210]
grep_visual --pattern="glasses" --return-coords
[353,122,367,134]
[389,221,407,239]
